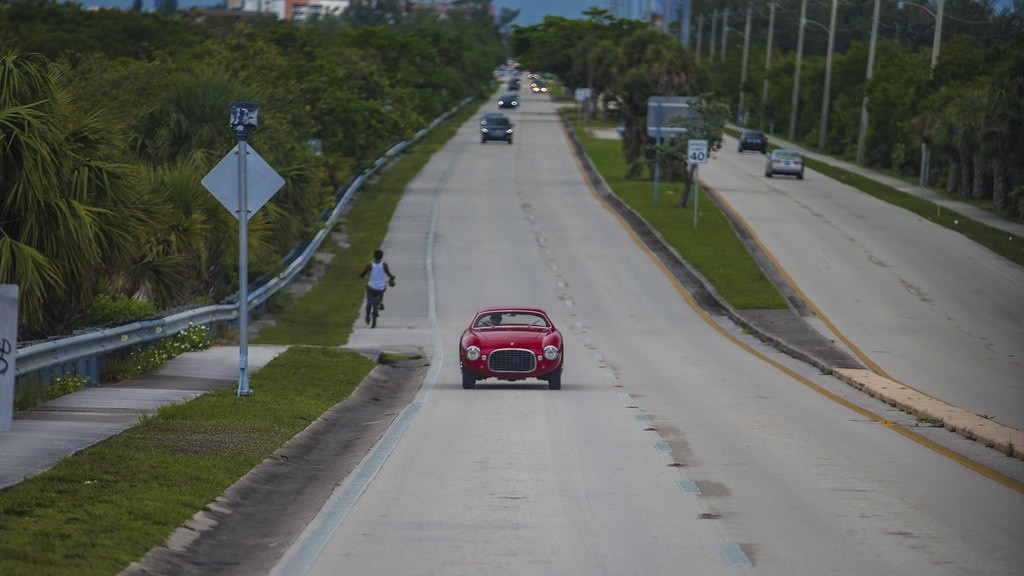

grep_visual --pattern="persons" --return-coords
[358,250,395,328]
[490,314,502,326]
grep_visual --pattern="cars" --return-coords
[480,112,515,144]
[459,306,565,390]
[764,148,805,179]
[495,59,548,110]
[737,130,769,153]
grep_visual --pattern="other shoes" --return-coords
[365,316,370,325]
[372,316,377,328]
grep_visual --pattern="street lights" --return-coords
[898,0,942,186]
[801,18,834,148]
[725,26,750,127]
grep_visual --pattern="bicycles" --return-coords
[363,277,395,328]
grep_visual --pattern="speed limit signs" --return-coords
[686,139,707,164]
